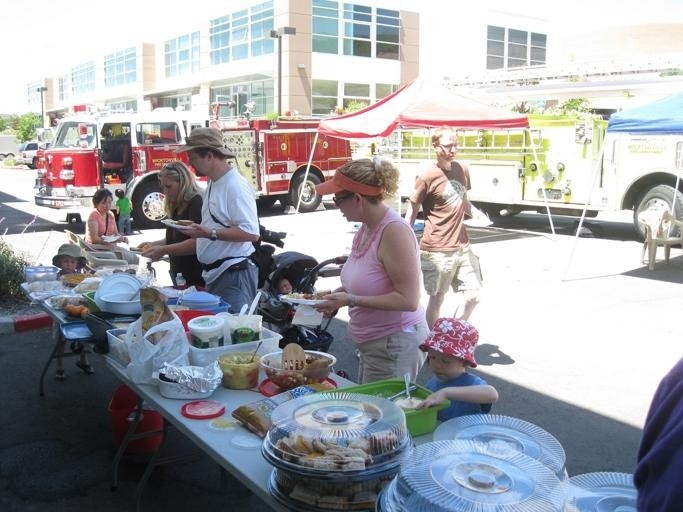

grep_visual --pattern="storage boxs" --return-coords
[166,294,232,318]
[84,308,132,337]
[174,325,281,376]
[107,325,131,367]
[84,248,125,271]
[82,290,100,314]
[316,371,453,440]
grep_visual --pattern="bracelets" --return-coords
[209,228,217,242]
[348,295,355,309]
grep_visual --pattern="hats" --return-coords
[52,244,86,269]
[172,127,238,157]
[418,317,480,368]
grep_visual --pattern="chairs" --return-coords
[636,202,682,270]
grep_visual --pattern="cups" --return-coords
[232,315,263,345]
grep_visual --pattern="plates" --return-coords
[160,218,199,232]
[127,248,147,255]
[280,292,327,307]
[179,293,220,308]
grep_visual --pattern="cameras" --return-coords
[259,224,286,248]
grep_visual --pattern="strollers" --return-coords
[259,253,349,356]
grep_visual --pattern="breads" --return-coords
[277,432,397,511]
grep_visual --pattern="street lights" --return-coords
[261,25,296,116]
[36,87,47,128]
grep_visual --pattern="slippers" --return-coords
[76,359,94,374]
[55,367,64,381]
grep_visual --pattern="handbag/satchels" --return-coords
[248,245,277,289]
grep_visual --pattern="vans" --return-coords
[20,142,47,169]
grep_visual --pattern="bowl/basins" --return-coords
[258,351,336,388]
[218,352,259,389]
[99,293,142,314]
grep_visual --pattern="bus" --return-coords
[370,47,683,247]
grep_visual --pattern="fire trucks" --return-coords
[33,93,354,228]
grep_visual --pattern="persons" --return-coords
[269,274,304,310]
[415,313,499,425]
[48,243,95,383]
[175,126,259,318]
[112,189,133,237]
[83,186,140,266]
[134,160,203,285]
[308,155,430,389]
[402,124,485,334]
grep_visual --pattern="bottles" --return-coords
[175,272,186,287]
